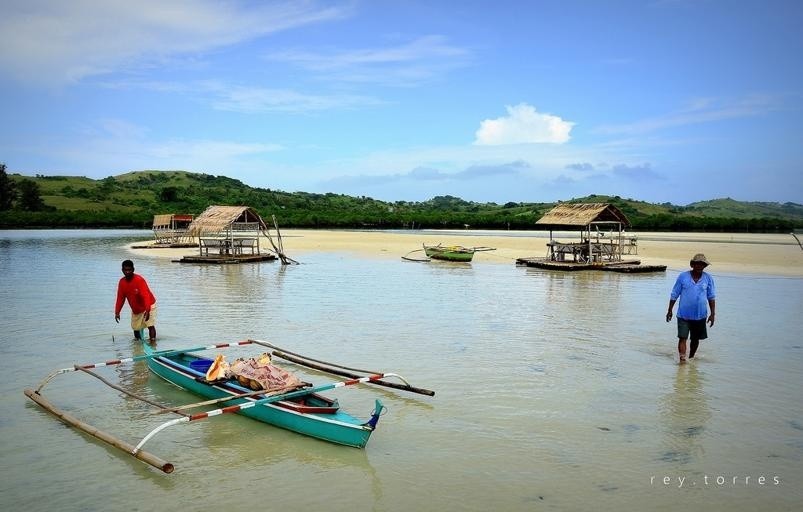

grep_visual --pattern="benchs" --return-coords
[547,242,620,263]
[155,231,193,243]
[200,237,255,256]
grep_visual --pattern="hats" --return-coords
[689,253,709,268]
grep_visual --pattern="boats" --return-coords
[422,242,475,262]
[423,257,473,277]
[138,324,383,450]
[137,368,383,502]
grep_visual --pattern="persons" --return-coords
[580,237,590,262]
[114,258,156,343]
[665,252,717,364]
